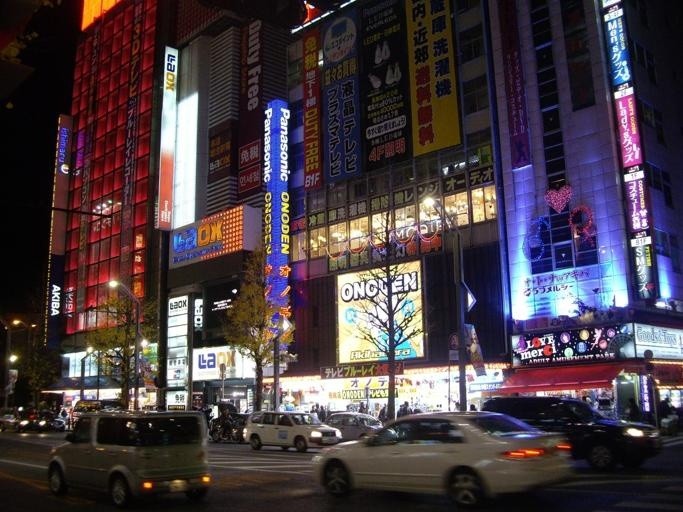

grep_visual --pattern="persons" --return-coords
[223,414,238,440]
[310,405,316,413]
[627,398,640,422]
[359,402,368,414]
[378,401,422,423]
[660,398,670,418]
[455,403,461,412]
[315,403,320,417]
[320,406,326,422]
[470,405,476,411]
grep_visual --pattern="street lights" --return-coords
[10,319,32,407]
[109,280,141,409]
[423,197,468,413]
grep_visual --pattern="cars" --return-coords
[1,414,66,432]
[311,410,574,508]
[209,412,250,443]
[323,412,396,442]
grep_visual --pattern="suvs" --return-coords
[242,412,343,452]
[479,396,661,470]
[47,410,214,506]
